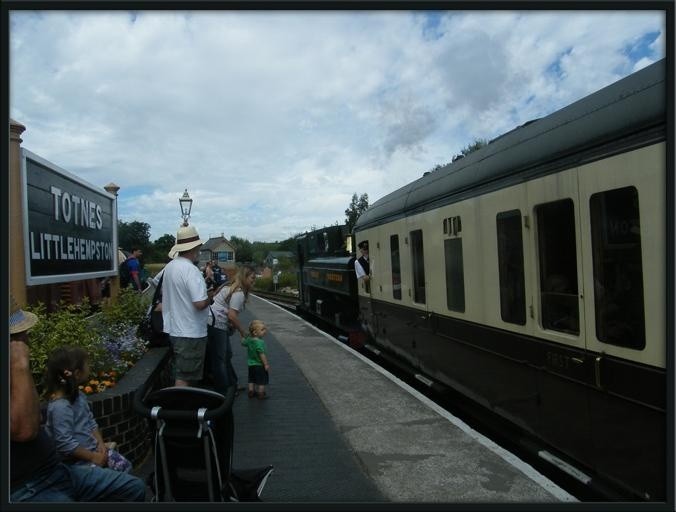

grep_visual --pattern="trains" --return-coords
[293,53,670,504]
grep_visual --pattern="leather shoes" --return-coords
[248,391,266,400]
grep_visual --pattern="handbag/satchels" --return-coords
[152,310,164,332]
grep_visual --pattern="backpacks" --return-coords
[120,258,136,288]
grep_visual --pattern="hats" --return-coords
[357,240,368,248]
[171,224,206,252]
[9,287,39,336]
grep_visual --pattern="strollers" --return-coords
[131,381,274,503]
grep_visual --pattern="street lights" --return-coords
[178,188,192,227]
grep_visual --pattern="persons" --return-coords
[119,246,143,294]
[150,226,270,398]
[354,239,372,291]
[10,293,145,502]
